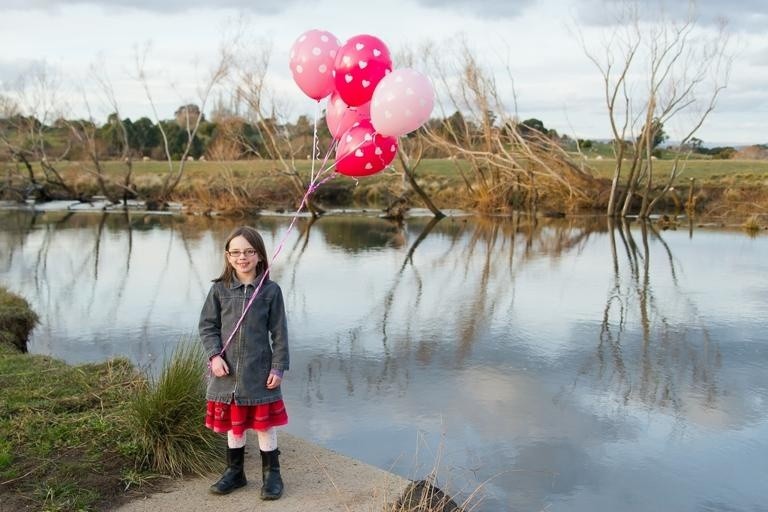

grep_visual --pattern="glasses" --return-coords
[227,249,258,257]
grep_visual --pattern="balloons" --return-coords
[289,28,435,178]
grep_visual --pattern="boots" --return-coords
[210,446,247,494]
[260,448,283,500]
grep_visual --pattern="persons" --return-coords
[198,226,290,500]
